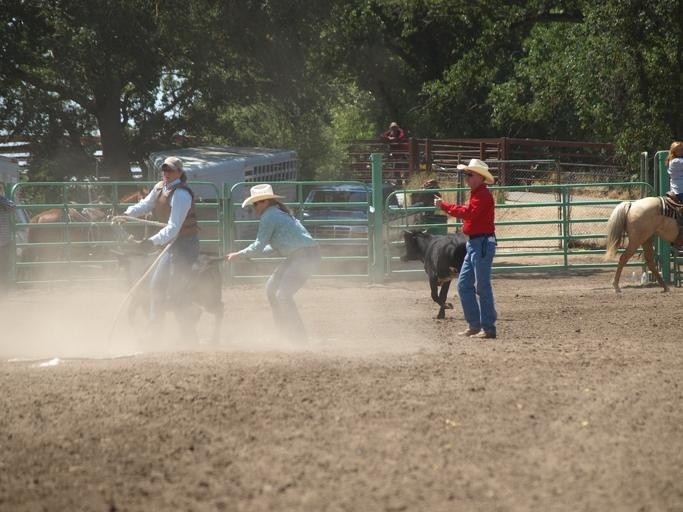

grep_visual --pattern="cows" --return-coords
[110,238,255,343]
[399,228,467,319]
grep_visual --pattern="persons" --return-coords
[433,158,498,339]
[378,121,411,186]
[0,182,16,281]
[224,183,320,344]
[668,142,682,205]
[112,156,201,339]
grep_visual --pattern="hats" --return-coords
[457,158,494,185]
[161,156,183,170]
[390,122,399,127]
[242,184,286,209]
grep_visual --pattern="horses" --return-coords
[601,195,683,293]
[409,177,443,217]
[14,185,156,282]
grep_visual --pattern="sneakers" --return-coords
[457,328,496,340]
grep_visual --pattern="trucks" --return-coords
[0,155,33,285]
[147,143,301,259]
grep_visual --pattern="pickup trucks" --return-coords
[295,184,434,246]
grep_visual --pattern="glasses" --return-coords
[463,173,473,177]
